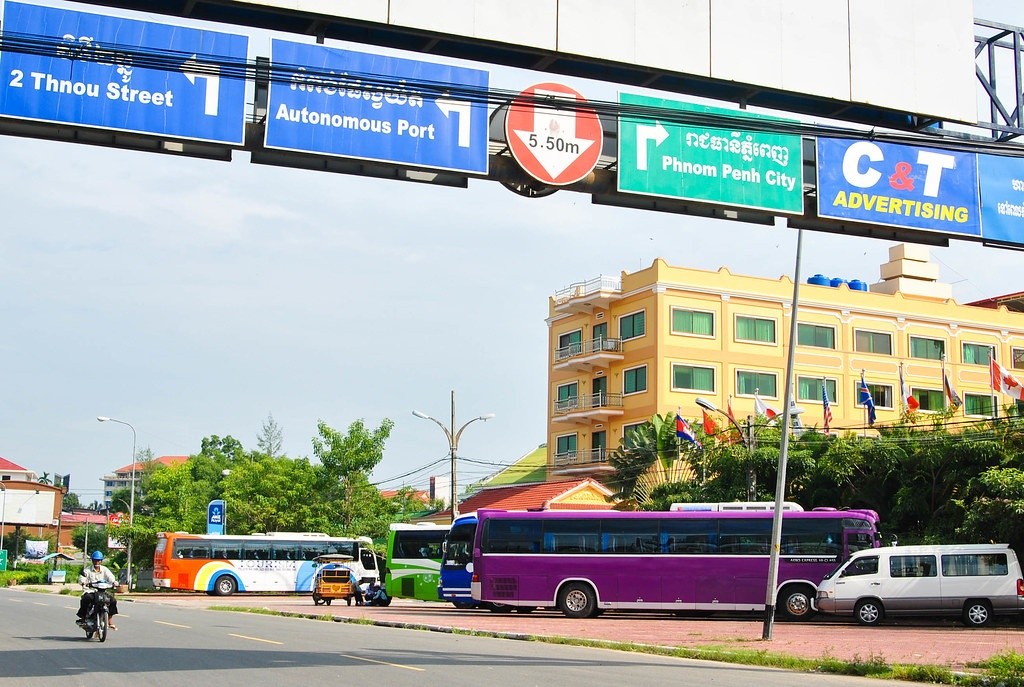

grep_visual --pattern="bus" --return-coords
[152,532,382,597]
[470,500,883,622]
[436,511,717,614]
[384,522,453,603]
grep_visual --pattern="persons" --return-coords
[350,580,391,606]
[77,551,119,629]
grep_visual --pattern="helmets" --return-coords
[91,551,104,560]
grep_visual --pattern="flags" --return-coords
[676,412,702,449]
[899,363,920,425]
[728,402,740,430]
[942,363,962,420]
[755,395,784,430]
[859,374,876,427]
[703,410,728,442]
[790,388,807,434]
[822,381,833,435]
[989,355,1024,401]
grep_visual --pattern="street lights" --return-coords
[694,397,804,500]
[412,389,495,525]
[96,415,138,587]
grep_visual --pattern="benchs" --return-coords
[290,550,306,560]
[192,549,208,558]
[253,549,269,559]
[304,551,320,560]
[323,570,351,583]
[215,551,227,559]
[179,549,193,558]
[275,549,290,559]
[226,550,240,559]
[246,550,259,560]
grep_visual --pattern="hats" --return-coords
[372,585,380,588]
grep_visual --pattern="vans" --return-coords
[814,543,1024,628]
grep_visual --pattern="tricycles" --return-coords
[312,553,355,607]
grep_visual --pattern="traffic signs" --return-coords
[0,0,250,148]
[264,38,490,176]
[616,89,804,216]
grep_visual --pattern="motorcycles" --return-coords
[78,581,122,643]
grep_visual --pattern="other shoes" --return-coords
[356,603,358,606]
[81,617,87,624]
[365,602,375,606]
[375,602,380,607]
[360,602,362,605]
[109,624,115,628]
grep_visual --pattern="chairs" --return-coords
[426,547,434,557]
[419,548,427,556]
